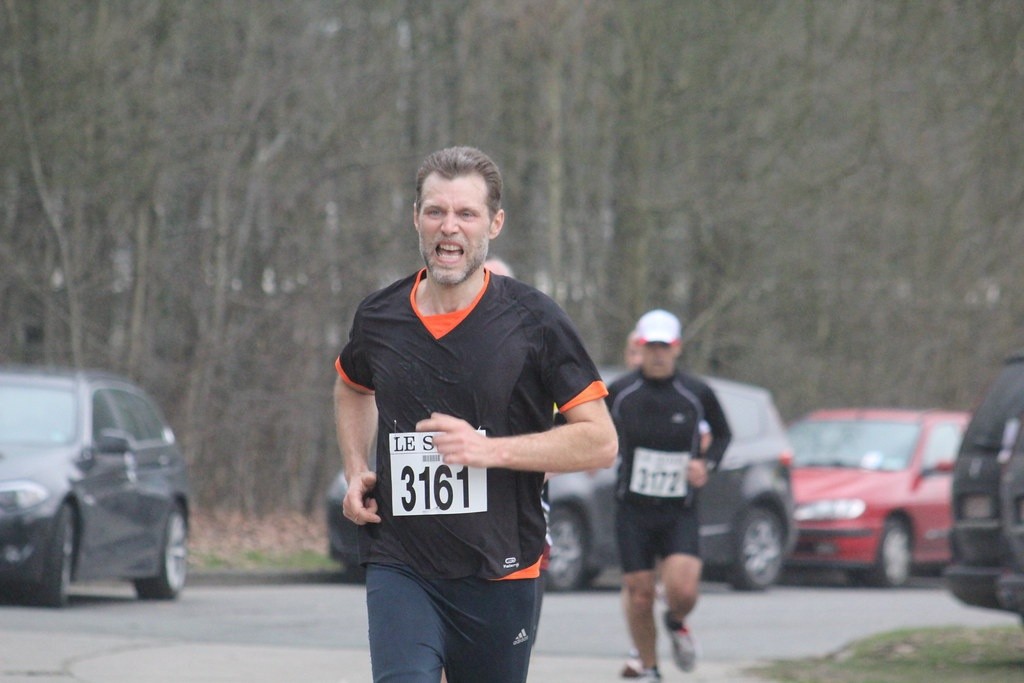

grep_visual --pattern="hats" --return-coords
[636,310,681,350]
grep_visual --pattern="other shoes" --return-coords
[640,668,662,683]
[621,647,647,677]
[663,610,696,672]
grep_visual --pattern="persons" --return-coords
[331,146,618,683]
[601,310,731,682]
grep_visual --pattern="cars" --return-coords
[785,409,972,588]
[0,364,189,610]
[939,352,1024,616]
[325,366,796,589]
